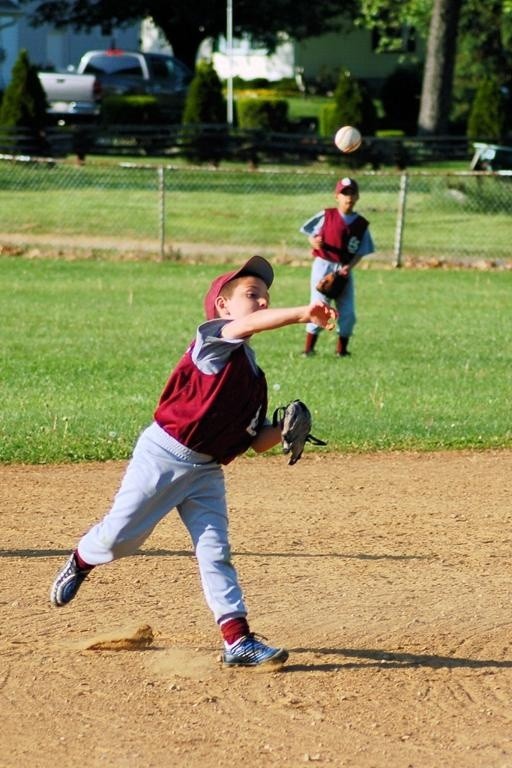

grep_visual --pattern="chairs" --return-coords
[223,632,289,665]
[50,552,91,608]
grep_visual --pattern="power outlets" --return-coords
[36,51,188,119]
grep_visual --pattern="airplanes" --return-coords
[281,399,311,467]
[316,269,350,301]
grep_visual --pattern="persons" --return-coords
[51,255,339,669]
[298,176,376,357]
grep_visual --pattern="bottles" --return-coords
[336,177,358,193]
[204,255,274,321]
